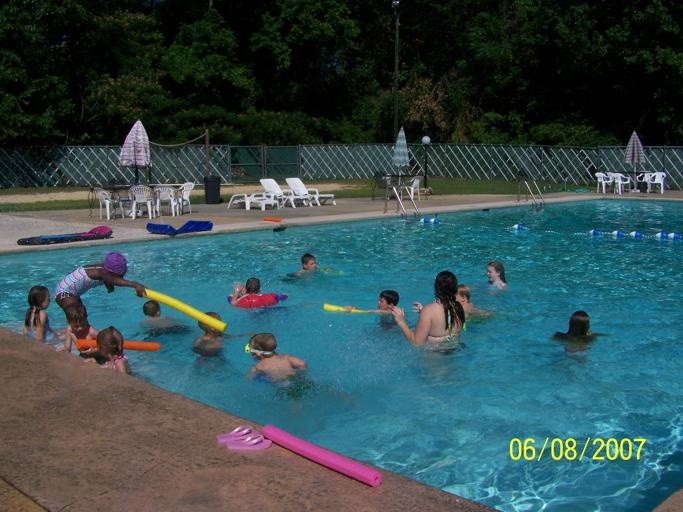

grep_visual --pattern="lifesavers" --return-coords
[227,293,289,308]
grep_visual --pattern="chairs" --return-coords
[594,170,666,196]
[227,178,338,214]
[97,181,195,220]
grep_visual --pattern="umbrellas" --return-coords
[624,130,646,190]
[392,126,409,185]
[118,120,151,183]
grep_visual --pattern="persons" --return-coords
[454,284,494,320]
[487,260,507,295]
[392,271,467,361]
[551,311,612,364]
[345,290,416,340]
[231,278,260,305]
[23,252,224,375]
[294,252,345,277]
[244,333,345,413]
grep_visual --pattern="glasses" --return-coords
[245,344,272,355]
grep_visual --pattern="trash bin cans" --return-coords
[204,175,221,204]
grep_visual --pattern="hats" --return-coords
[104,253,127,276]
